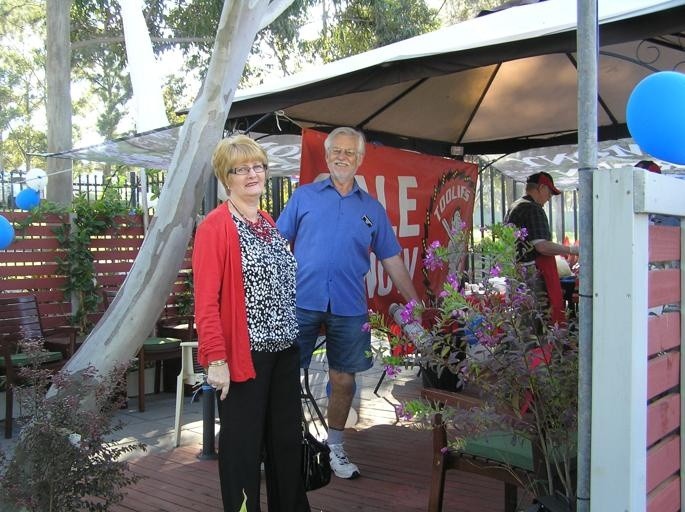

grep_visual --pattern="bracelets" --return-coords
[207,359,227,367]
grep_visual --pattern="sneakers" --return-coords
[325,441,361,480]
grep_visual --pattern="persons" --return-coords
[272,128,430,479]
[503,171,581,326]
[192,133,317,510]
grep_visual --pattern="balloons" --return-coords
[0,215,14,250]
[14,188,39,210]
[25,168,47,192]
[625,71,685,165]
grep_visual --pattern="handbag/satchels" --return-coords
[299,410,331,493]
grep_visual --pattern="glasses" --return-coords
[226,163,268,180]
[328,146,359,157]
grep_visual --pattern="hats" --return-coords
[526,170,564,196]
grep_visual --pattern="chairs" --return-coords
[154,297,205,401]
[0,294,75,440]
[102,290,183,414]
[420,386,578,512]
[173,341,204,448]
[45,334,86,390]
